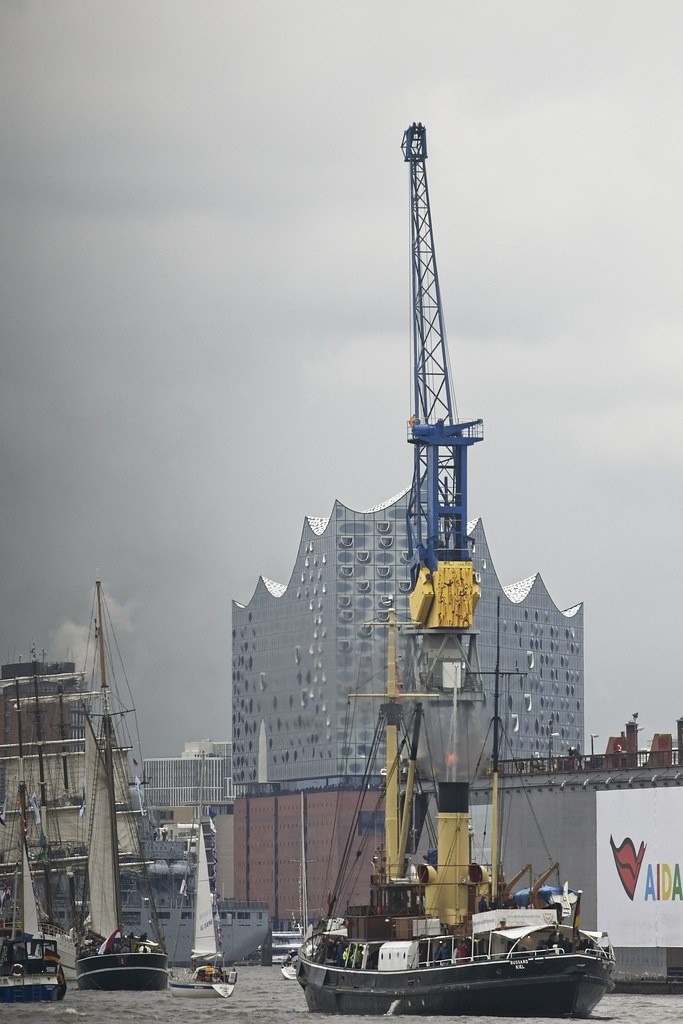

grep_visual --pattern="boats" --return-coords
[514,881,583,917]
[270,930,303,964]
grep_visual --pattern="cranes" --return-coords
[382,120,502,931]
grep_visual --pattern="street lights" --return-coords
[591,734,599,754]
[635,726,646,768]
[549,732,561,772]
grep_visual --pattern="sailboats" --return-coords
[167,751,238,998]
[294,595,618,1020]
[280,790,300,981]
[0,579,268,1004]
